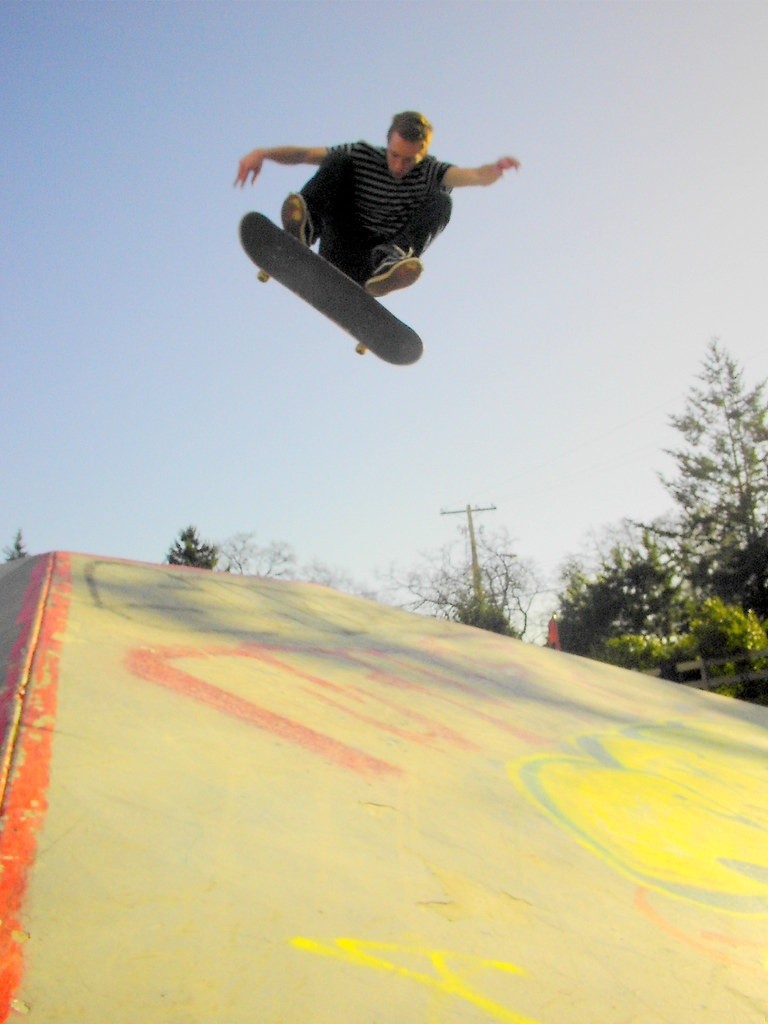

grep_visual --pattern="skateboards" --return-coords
[237,212,424,367]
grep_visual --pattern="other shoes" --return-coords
[365,244,423,298]
[281,193,314,247]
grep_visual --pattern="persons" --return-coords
[233,110,521,298]
